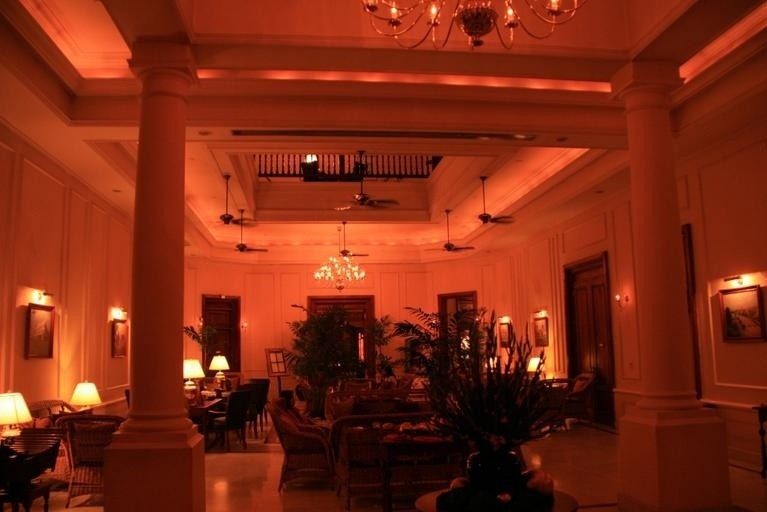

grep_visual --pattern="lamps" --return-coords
[182,355,230,398]
[0,383,103,437]
[360,0,585,52]
[484,355,544,372]
[312,227,368,291]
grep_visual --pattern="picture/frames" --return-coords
[532,317,550,347]
[23,303,56,358]
[497,323,513,346]
[110,319,129,358]
[718,284,767,343]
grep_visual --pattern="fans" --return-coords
[422,209,476,252]
[214,174,268,253]
[338,221,370,257]
[332,150,400,212]
[477,176,516,225]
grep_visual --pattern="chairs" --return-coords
[533,369,600,427]
[0,399,127,512]
[124,389,130,408]
[206,378,269,451]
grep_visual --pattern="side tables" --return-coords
[185,398,225,443]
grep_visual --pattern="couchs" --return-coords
[264,378,470,512]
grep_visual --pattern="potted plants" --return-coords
[392,301,572,491]
